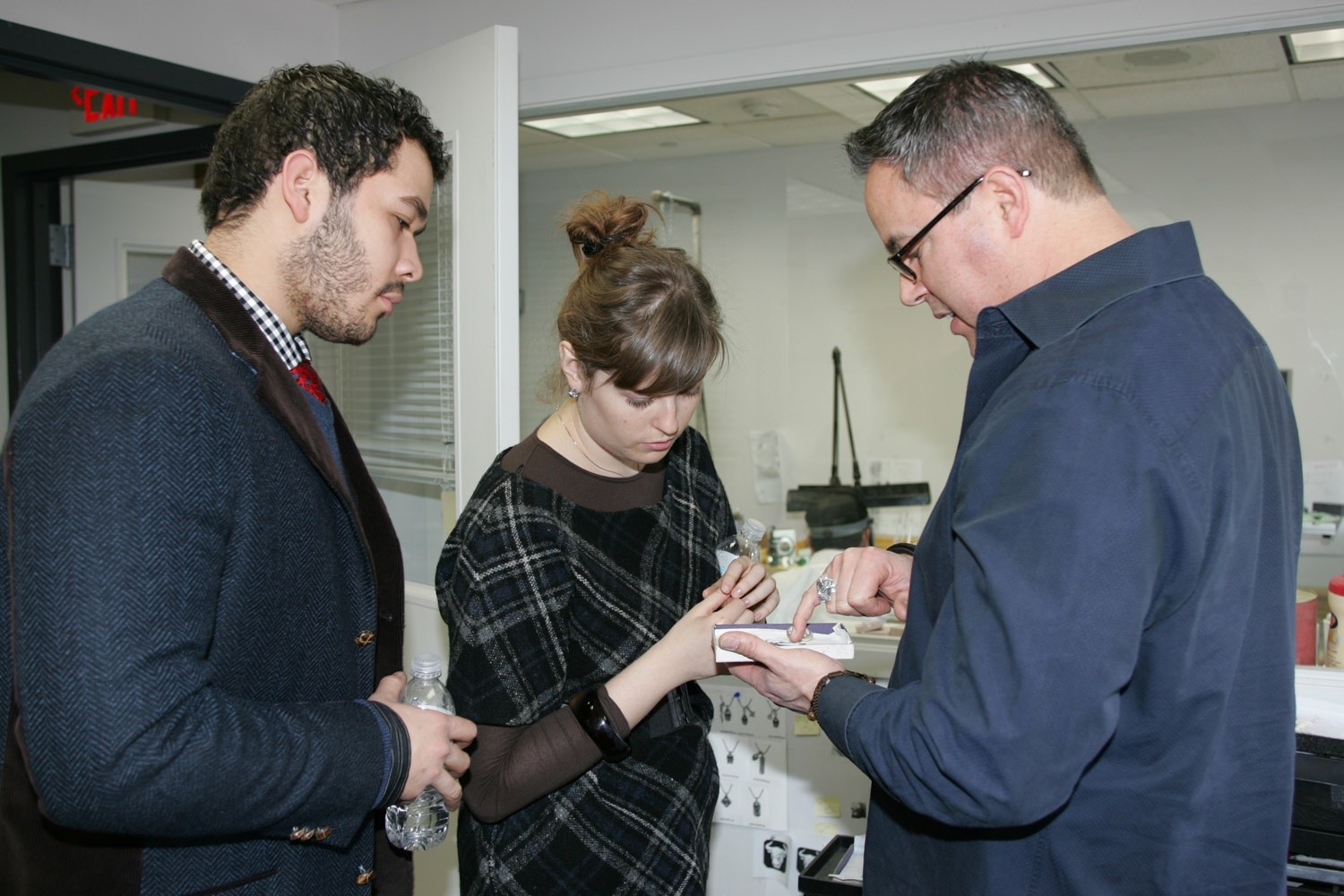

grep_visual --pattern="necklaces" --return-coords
[558,416,625,478]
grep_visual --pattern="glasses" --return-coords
[289,356,328,405]
[887,168,1032,283]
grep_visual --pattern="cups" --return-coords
[1295,584,1319,666]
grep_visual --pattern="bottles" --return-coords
[1326,574,1344,668]
[714,517,766,578]
[385,652,454,853]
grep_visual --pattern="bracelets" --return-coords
[808,670,875,722]
[577,685,629,763]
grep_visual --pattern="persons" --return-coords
[719,62,1304,895]
[434,201,782,895]
[0,64,478,896]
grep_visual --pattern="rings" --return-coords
[815,578,837,600]
